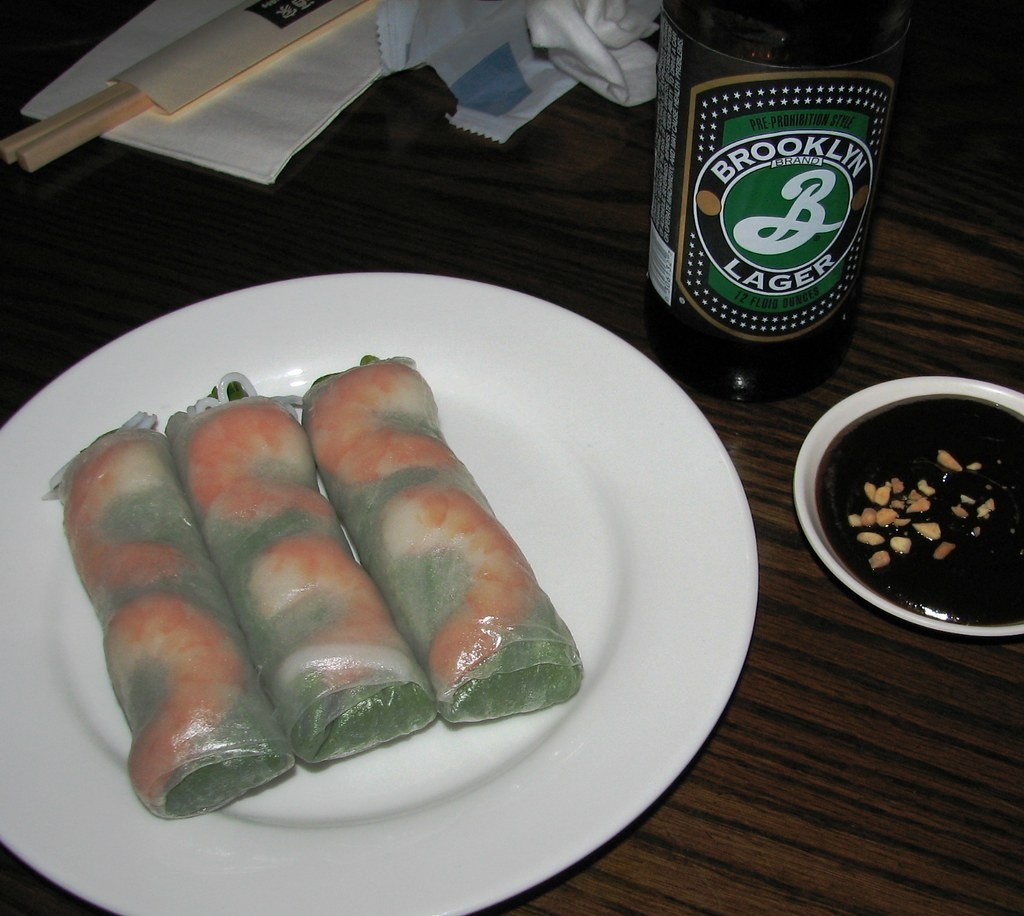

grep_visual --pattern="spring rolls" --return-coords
[49,353,584,823]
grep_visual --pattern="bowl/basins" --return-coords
[794,380,1024,638]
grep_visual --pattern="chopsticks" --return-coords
[1,78,158,174]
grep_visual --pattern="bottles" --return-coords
[631,0,910,412]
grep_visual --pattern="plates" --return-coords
[0,268,763,915]
